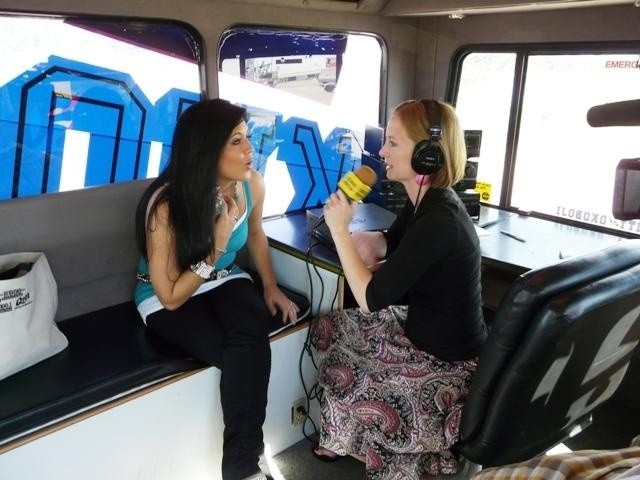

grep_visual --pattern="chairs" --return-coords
[441,237,640,480]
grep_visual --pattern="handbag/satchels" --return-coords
[0,251,69,381]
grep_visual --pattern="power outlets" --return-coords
[291,397,306,427]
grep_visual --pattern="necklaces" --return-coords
[213,184,242,215]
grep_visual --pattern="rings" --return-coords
[234,215,239,221]
[290,301,293,306]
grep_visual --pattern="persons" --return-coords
[310,97,491,479]
[131,97,302,480]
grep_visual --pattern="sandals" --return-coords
[312,446,337,463]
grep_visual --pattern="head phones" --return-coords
[411,99,445,175]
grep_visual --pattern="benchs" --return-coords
[1,178,323,480]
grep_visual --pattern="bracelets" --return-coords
[211,246,225,253]
[189,261,216,280]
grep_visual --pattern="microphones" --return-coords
[319,165,378,224]
